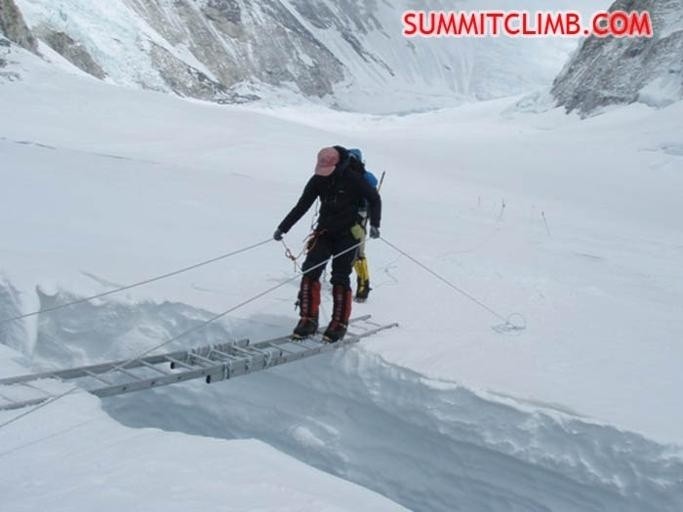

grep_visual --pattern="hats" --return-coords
[314,146,340,177]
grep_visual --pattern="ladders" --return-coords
[0,315,400,414]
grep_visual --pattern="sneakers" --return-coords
[323,321,347,342]
[292,318,318,339]
[353,280,369,302]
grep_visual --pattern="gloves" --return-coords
[369,227,380,239]
[274,230,283,240]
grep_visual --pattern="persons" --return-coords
[348,149,380,302]
[274,145,381,341]
